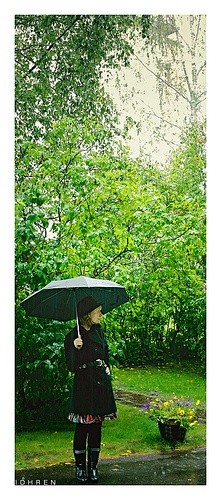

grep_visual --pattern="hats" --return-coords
[78,296,101,317]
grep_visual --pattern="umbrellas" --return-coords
[19,276,130,346]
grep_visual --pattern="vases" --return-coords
[158,420,186,442]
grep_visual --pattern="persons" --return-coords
[63,294,118,483]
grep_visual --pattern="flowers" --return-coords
[138,390,201,429]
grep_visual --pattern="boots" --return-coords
[87,447,101,482]
[74,450,88,482]
